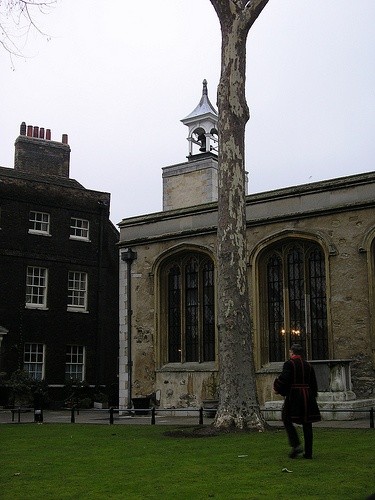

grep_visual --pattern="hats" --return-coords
[291,343,303,355]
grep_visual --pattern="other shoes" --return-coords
[303,454,312,459]
[288,447,303,458]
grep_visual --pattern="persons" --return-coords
[273,344,321,459]
